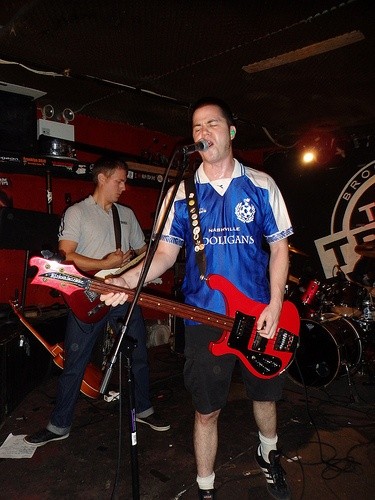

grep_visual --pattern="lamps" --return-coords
[37,103,75,143]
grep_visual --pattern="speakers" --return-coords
[0,90,37,156]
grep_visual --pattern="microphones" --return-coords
[336,266,351,281]
[180,140,208,155]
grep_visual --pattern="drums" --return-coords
[292,275,332,316]
[286,312,363,390]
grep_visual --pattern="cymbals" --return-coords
[354,241,375,256]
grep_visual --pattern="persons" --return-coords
[100,99,294,500]
[24,158,171,446]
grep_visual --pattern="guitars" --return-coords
[58,251,147,323]
[9,297,105,400]
[30,256,301,379]
[321,280,374,330]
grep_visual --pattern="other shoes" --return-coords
[24,428,69,446]
[136,412,170,431]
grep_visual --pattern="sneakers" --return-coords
[197,479,216,500]
[254,442,293,500]
